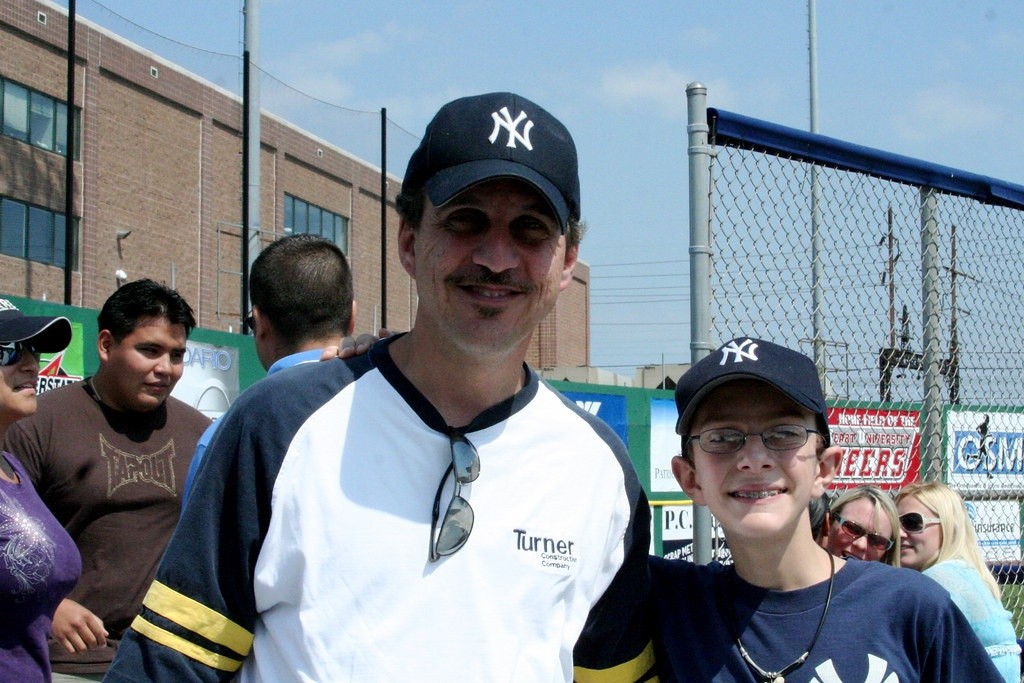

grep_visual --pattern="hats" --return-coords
[400,91,583,235]
[0,299,77,355]
[670,337,829,436]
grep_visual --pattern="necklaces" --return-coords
[727,545,835,683]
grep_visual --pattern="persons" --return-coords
[5,277,214,683]
[0,300,82,683]
[179,233,357,519]
[322,328,1006,683]
[805,482,1023,683]
[101,90,660,683]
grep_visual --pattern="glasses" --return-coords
[244,309,254,329]
[899,510,942,534]
[697,422,811,455]
[833,514,895,554]
[1,343,43,366]
[427,425,481,564]
